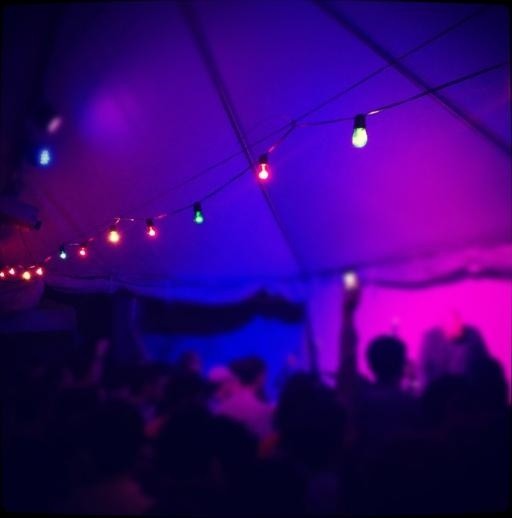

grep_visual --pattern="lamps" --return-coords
[258,153,270,180]
[353,114,368,149]
[193,200,203,224]
[59,245,65,259]
[146,218,156,236]
[79,243,87,256]
[110,224,117,243]
